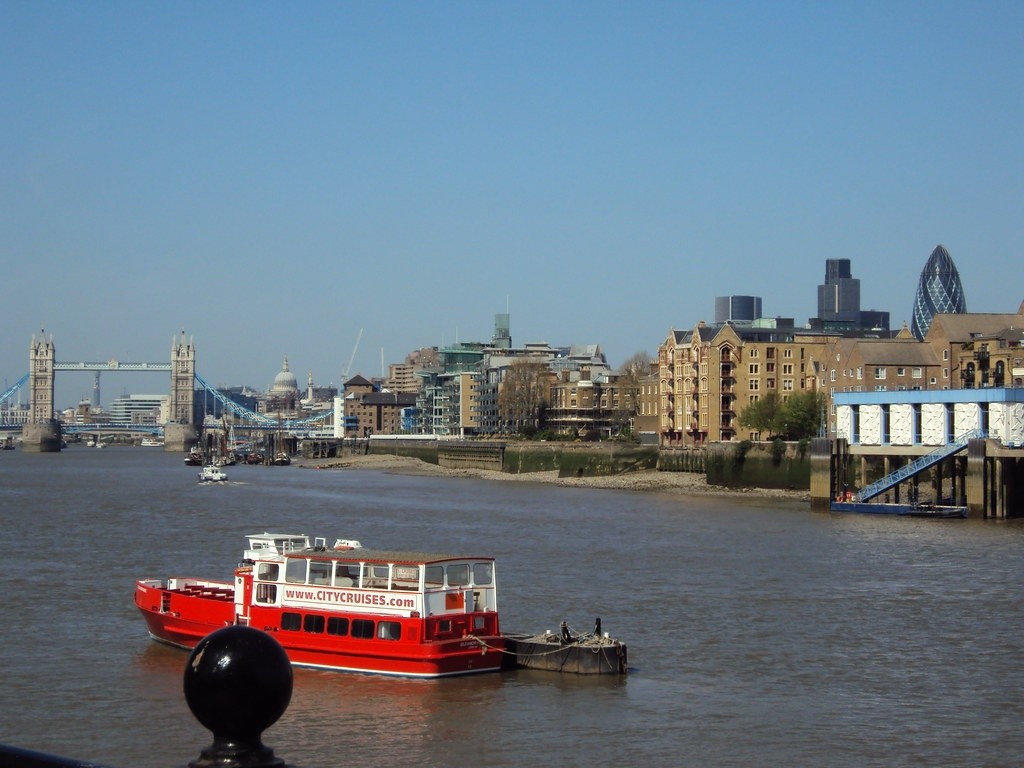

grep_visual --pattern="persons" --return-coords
[338,562,349,577]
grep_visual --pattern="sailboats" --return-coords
[272,411,290,465]
[184,421,237,468]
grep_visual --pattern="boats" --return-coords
[246,454,263,465]
[198,464,228,482]
[134,532,505,679]
[87,441,95,446]
[141,438,164,447]
[96,443,106,448]
